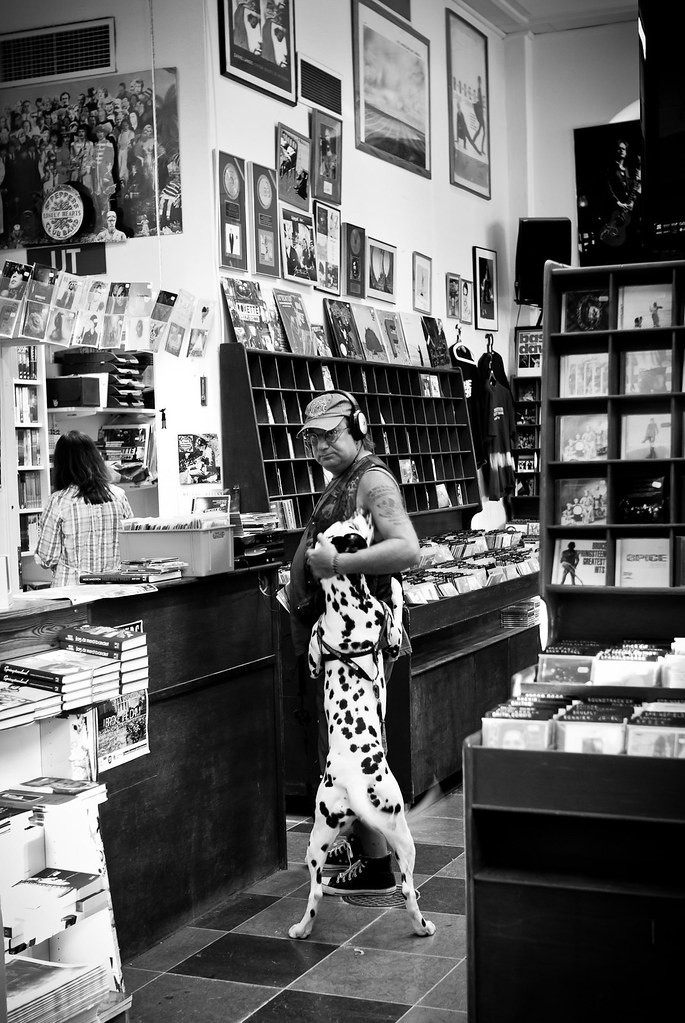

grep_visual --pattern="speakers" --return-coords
[514,216,571,308]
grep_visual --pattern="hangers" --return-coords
[452,324,497,382]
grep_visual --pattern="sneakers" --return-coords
[323,833,362,870]
[320,852,396,896]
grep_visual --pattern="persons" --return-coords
[462,281,472,322]
[179,437,219,482]
[288,392,422,897]
[33,430,134,589]
[284,230,315,276]
[0,263,104,347]
[603,138,641,230]
[0,78,182,247]
[104,284,208,356]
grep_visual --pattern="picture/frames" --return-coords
[313,198,341,297]
[472,245,498,332]
[515,329,542,378]
[460,278,473,326]
[246,159,281,278]
[279,202,321,287]
[445,6,492,201]
[445,272,462,319]
[217,0,298,108]
[412,251,433,315]
[367,236,398,304]
[276,121,311,212]
[311,107,342,207]
[213,147,248,273]
[352,0,432,181]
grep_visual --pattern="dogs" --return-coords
[287,515,435,938]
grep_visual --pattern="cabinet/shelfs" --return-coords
[0,345,158,557]
[0,711,130,1023]
[535,258,684,646]
[219,341,483,531]
[462,730,685,1023]
[508,374,541,521]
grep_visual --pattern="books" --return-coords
[79,569,184,583]
[239,511,279,534]
[20,514,41,552]
[14,427,41,466]
[17,470,42,508]
[484,632,685,758]
[16,344,38,380]
[15,386,38,423]
[270,498,297,528]
[0,776,108,837]
[2,867,106,951]
[548,282,685,586]
[0,623,148,730]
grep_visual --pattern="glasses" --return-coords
[306,426,350,446]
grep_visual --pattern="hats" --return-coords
[297,391,355,436]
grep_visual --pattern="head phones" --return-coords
[315,390,367,441]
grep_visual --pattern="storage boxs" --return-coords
[46,377,100,406]
[117,523,236,577]
[481,653,685,758]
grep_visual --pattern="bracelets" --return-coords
[332,553,341,574]
